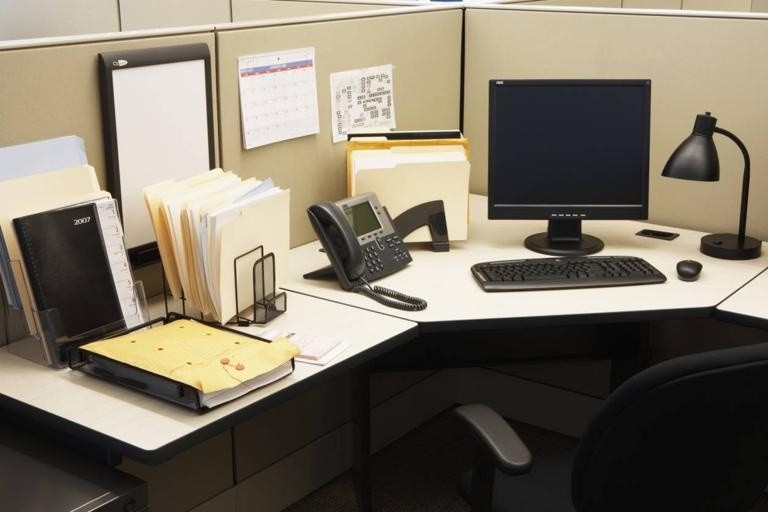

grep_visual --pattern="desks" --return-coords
[0,189,768,512]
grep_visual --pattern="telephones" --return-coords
[305,191,428,313]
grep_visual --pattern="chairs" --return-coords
[454,340,768,511]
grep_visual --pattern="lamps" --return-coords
[661,112,763,260]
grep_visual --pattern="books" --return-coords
[0,136,144,362]
[142,168,292,326]
[78,317,297,409]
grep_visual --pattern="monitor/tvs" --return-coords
[486,79,652,257]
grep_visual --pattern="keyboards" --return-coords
[470,257,667,292]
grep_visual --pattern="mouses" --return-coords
[676,259,701,282]
